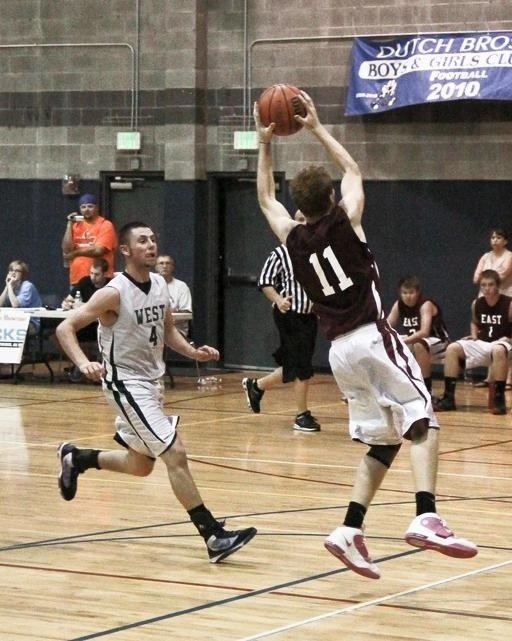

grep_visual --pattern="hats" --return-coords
[78,193,97,205]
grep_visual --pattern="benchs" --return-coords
[432,358,511,381]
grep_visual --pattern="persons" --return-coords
[61,193,116,293]
[0,259,43,336]
[61,258,116,310]
[386,273,452,398]
[58,223,257,563]
[242,208,321,431]
[429,270,512,415]
[154,255,193,340]
[250,88,478,579]
[472,227,512,296]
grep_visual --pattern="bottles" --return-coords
[75,289,83,307]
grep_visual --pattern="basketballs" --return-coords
[257,83,307,137]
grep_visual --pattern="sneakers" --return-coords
[403,512,478,559]
[323,525,381,580]
[491,391,507,414]
[201,523,257,564]
[293,411,320,432]
[241,377,262,414]
[435,392,457,411]
[55,441,80,501]
[69,350,96,383]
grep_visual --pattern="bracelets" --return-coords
[256,139,273,148]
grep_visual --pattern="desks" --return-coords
[0,308,193,387]
